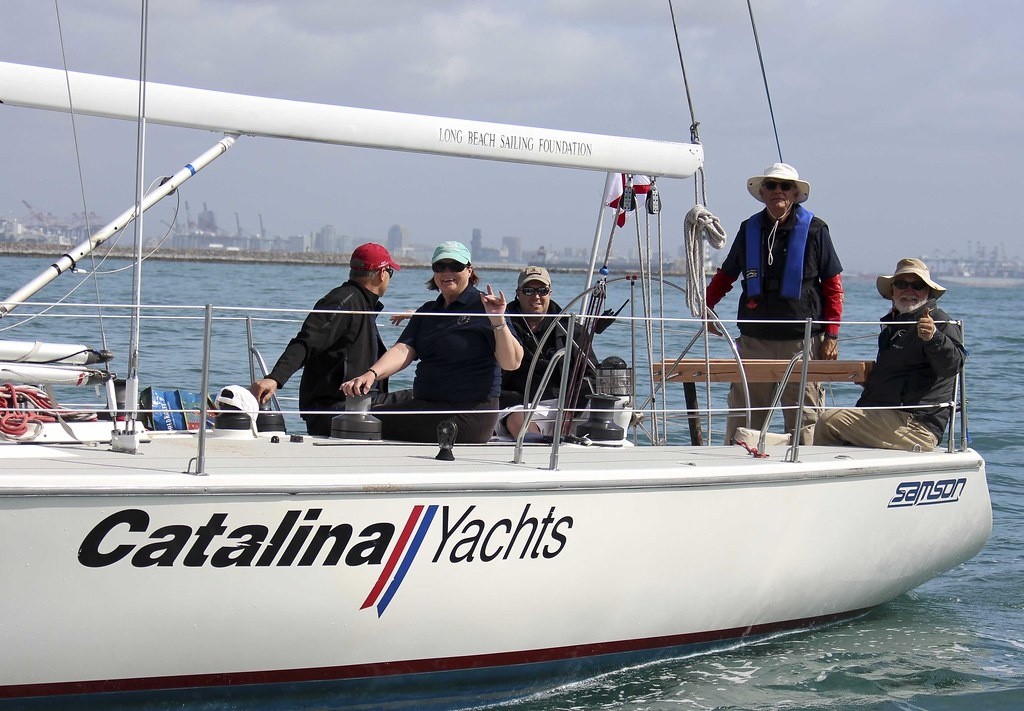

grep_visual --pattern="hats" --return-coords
[876,258,947,301]
[747,163,810,203]
[350,242,400,273]
[432,240,471,265]
[518,265,551,289]
[214,385,260,439]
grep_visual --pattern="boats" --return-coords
[0,0,995,711]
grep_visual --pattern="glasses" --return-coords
[382,267,394,278]
[759,180,797,192]
[432,261,470,273]
[890,279,925,291]
[520,287,551,296]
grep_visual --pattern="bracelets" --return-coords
[363,368,377,383]
[824,333,838,339]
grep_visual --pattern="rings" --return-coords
[921,328,923,333]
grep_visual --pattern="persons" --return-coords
[339,241,524,444]
[390,265,601,442]
[700,163,845,443]
[800,257,969,452]
[251,242,415,436]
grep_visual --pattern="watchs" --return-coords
[491,319,506,330]
